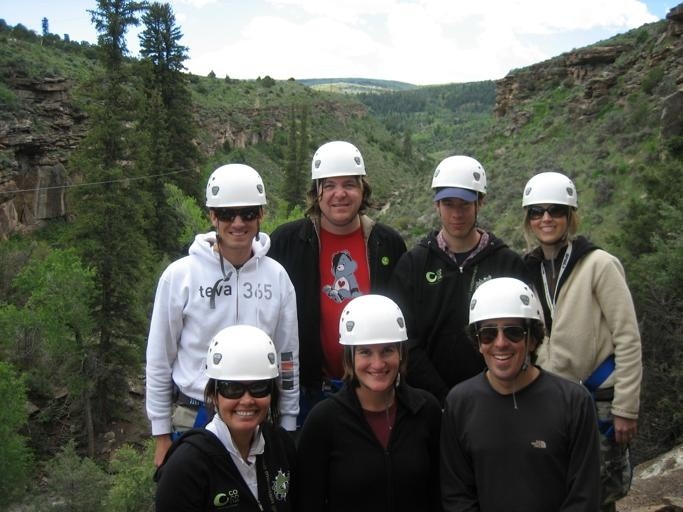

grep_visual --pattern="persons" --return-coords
[143,163,302,467]
[298,294,442,511]
[267,141,407,435]
[152,324,297,511]
[437,276,603,511]
[519,170,646,509]
[388,155,531,405]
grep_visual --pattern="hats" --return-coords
[433,187,481,203]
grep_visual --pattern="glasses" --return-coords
[526,204,569,220]
[212,208,261,221]
[216,381,272,399]
[478,326,527,345]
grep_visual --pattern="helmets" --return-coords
[310,141,367,180]
[205,163,267,207]
[468,277,543,325]
[430,155,486,195]
[204,325,280,381]
[522,171,579,209]
[337,293,410,346]
